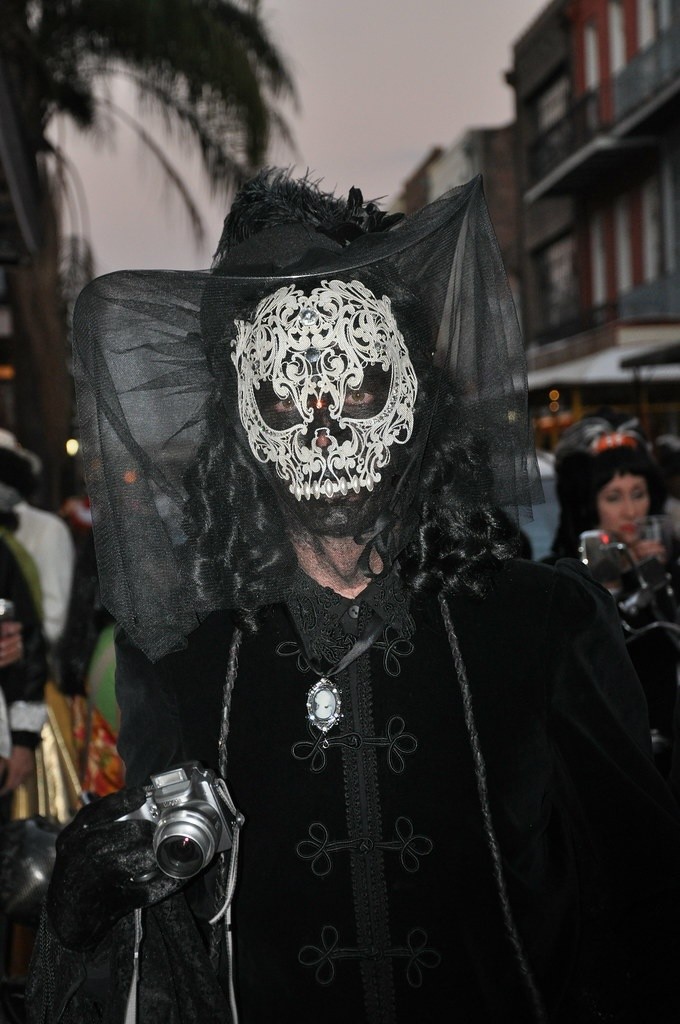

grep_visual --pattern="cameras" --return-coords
[117,756,234,882]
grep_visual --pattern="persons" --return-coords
[1,175,680,1024]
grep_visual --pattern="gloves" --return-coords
[46,788,188,949]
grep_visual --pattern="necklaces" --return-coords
[271,586,390,736]
